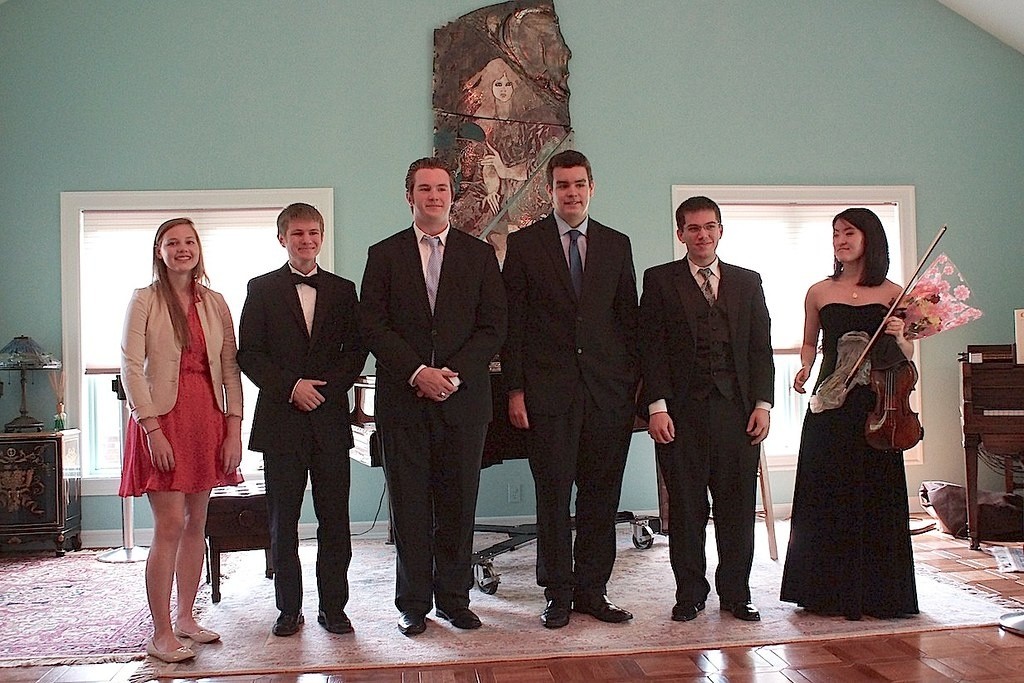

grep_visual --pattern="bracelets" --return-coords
[145,427,160,435]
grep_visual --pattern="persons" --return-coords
[639,196,775,620]
[235,202,370,635]
[496,149,640,627]
[780,208,920,621]
[119,219,245,662]
[361,157,509,633]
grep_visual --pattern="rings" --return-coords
[440,391,445,398]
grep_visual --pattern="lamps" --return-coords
[0,334,65,433]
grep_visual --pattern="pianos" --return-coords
[348,356,668,595]
[958,343,1024,551]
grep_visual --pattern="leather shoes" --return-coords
[719,601,761,621]
[272,608,305,636]
[671,600,705,621]
[146,637,196,663]
[317,609,353,634]
[397,610,427,637]
[173,624,221,644]
[436,608,483,629]
[573,594,634,623]
[540,599,572,629]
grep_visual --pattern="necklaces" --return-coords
[853,292,858,299]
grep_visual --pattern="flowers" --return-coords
[809,254,984,413]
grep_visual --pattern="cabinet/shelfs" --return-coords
[0,428,81,555]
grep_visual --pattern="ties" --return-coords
[566,230,584,303]
[699,269,716,307]
[423,236,443,317]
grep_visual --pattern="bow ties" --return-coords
[291,273,318,289]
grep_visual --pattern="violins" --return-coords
[863,296,924,452]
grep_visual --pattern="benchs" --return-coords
[205,477,274,602]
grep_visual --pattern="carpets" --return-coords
[0,547,240,668]
[132,505,1024,681]
